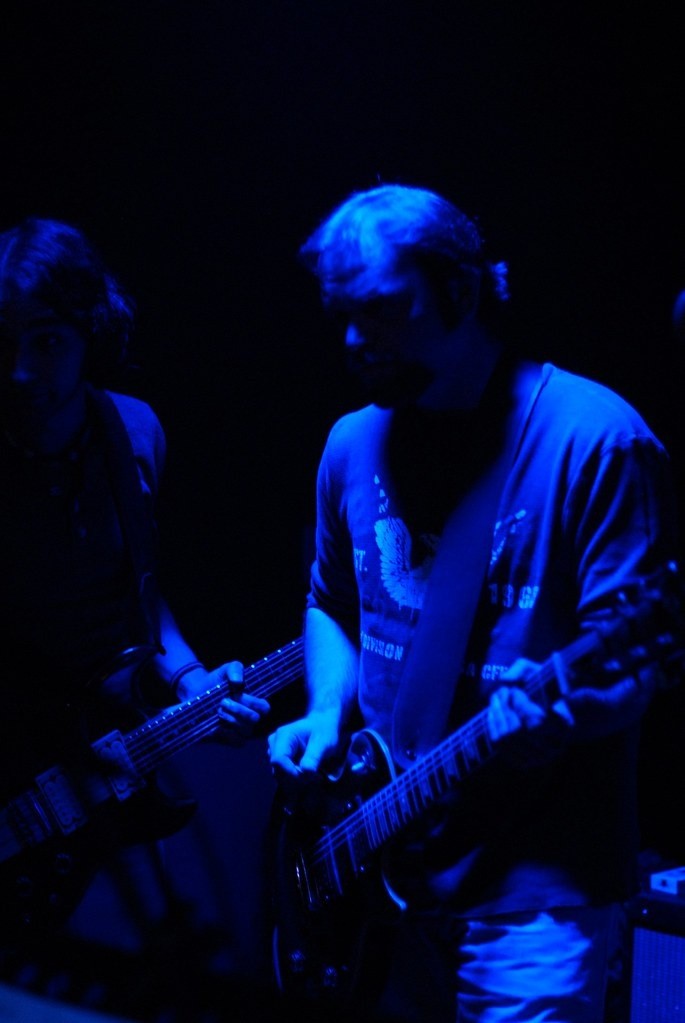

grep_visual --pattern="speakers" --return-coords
[603,890,685,1023]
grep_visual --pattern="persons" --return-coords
[0,214,280,986]
[272,183,674,1023]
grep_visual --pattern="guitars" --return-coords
[0,630,306,970]
[273,570,684,1006]
